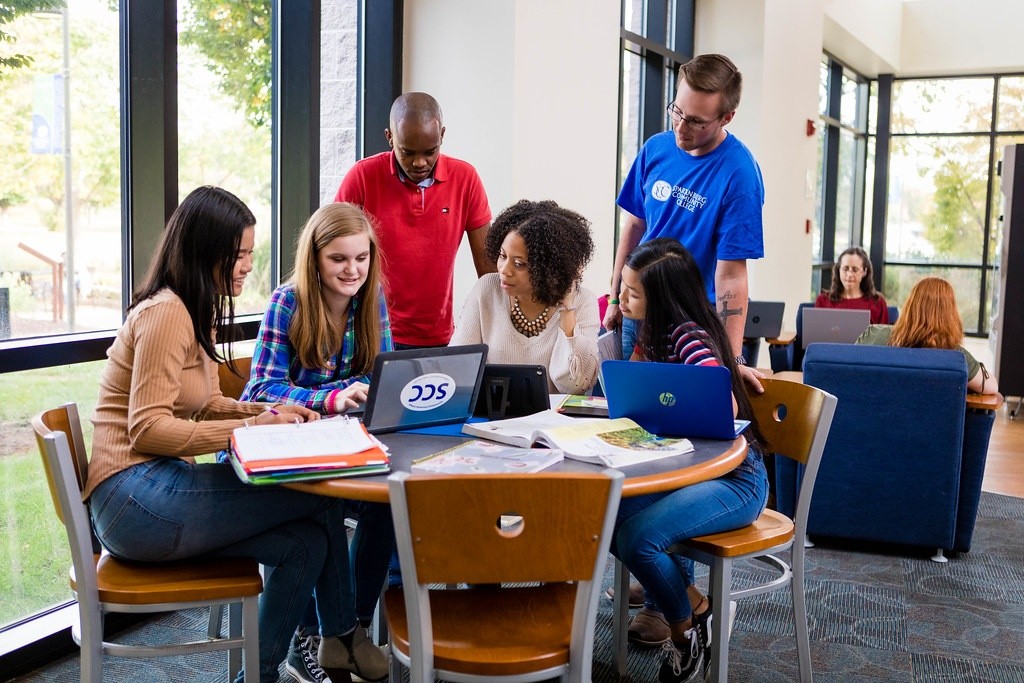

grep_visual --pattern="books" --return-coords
[411,441,564,475]
[462,409,695,469]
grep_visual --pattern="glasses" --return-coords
[667,99,725,132]
[840,267,864,276]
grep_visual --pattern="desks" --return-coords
[278,393,749,588]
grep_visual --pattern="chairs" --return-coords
[383,468,624,683]
[31,402,265,683]
[613,379,837,683]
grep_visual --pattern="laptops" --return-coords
[601,359,752,440]
[744,301,785,338]
[320,343,489,435]
[802,307,871,350]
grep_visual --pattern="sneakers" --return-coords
[657,633,703,683]
[693,596,739,681]
[286,631,332,683]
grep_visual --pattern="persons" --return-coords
[447,200,600,394]
[602,54,766,645]
[333,92,498,351]
[216,202,395,683]
[814,247,889,324]
[84,184,389,683]
[854,277,998,395]
[609,238,770,683]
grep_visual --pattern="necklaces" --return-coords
[510,296,549,338]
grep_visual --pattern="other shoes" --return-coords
[606,581,646,607]
[628,608,672,643]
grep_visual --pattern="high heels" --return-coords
[317,628,390,683]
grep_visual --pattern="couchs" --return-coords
[775,341,1004,563]
[765,303,900,373]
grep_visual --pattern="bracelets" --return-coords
[557,306,576,312]
[734,356,746,364]
[328,388,341,415]
[608,300,618,304]
